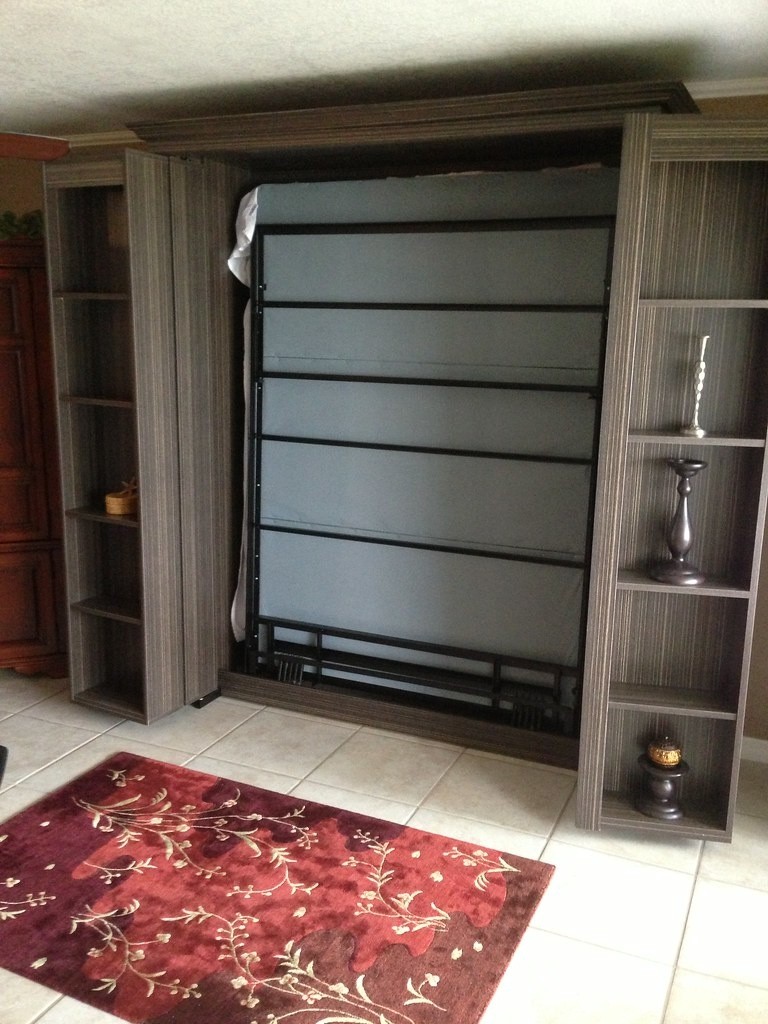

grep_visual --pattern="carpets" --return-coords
[0,751,560,1024]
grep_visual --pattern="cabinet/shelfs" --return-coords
[576,110,768,843]
[34,149,237,726]
